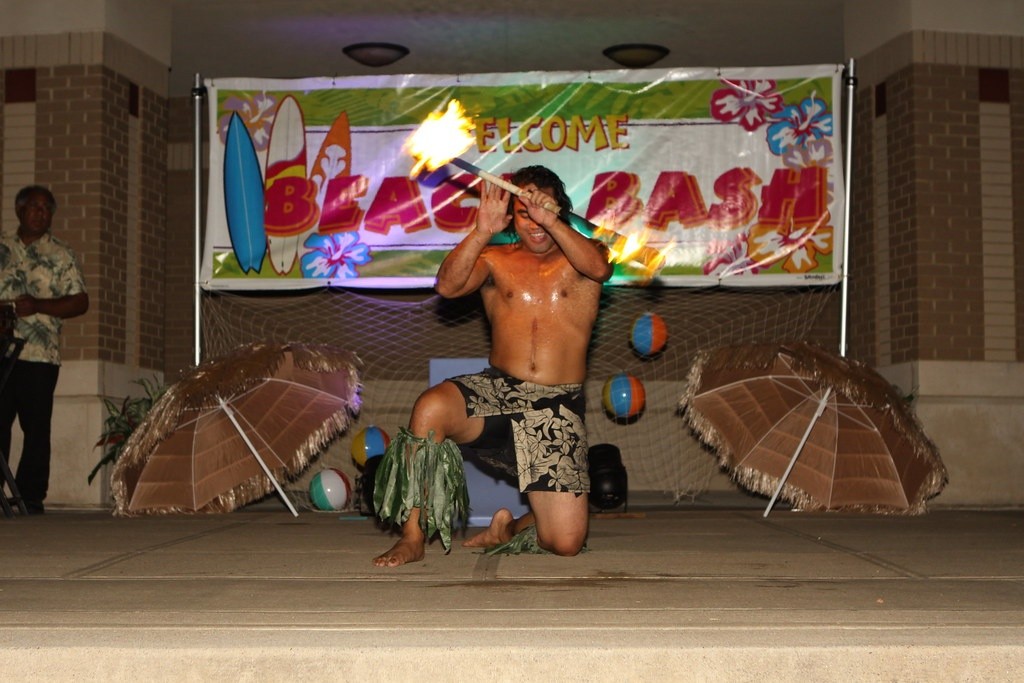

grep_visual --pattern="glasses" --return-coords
[20,202,56,215]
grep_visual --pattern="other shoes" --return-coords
[24,499,44,519]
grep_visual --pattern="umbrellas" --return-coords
[681,336,949,519]
[109,335,366,518]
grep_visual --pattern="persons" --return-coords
[0,183,90,515]
[372,165,614,568]
